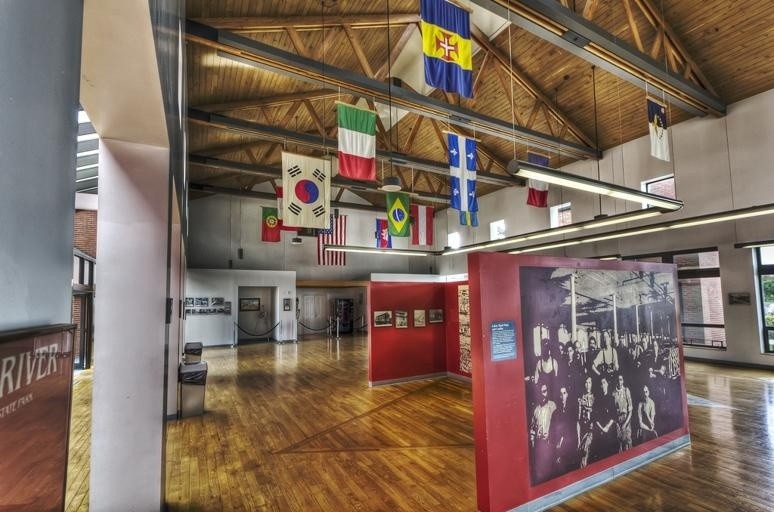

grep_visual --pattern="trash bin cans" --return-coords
[184,342,202,363]
[179,361,208,417]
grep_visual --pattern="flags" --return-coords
[420,1,475,100]
[409,203,434,247]
[374,217,392,249]
[334,102,377,184]
[646,96,671,163]
[275,186,304,232]
[446,132,478,213]
[315,213,347,267]
[458,208,479,228]
[525,151,549,209]
[280,151,330,229]
[260,206,280,243]
[384,191,410,238]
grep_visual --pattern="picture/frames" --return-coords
[239,297,260,312]
[0,323,79,512]
[372,306,445,329]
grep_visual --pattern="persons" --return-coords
[527,331,681,484]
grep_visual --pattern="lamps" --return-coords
[226,130,246,270]
[322,0,774,257]
